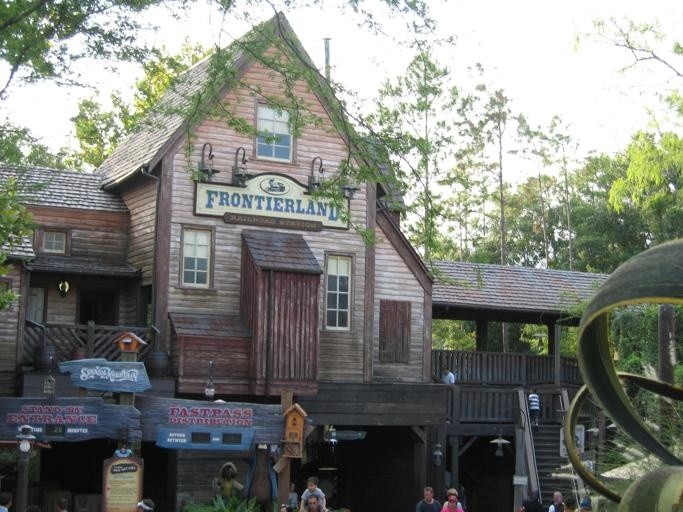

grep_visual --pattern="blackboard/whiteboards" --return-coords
[103,458,144,512]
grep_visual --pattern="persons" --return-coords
[279,477,327,512]
[416,486,441,512]
[137,498,155,512]
[441,364,455,383]
[56,497,69,512]
[528,386,540,431]
[516,491,592,512]
[440,488,464,512]
[215,462,244,502]
[0,492,12,512]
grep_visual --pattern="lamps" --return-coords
[205,361,216,400]
[433,443,443,467]
[303,157,360,199]
[198,141,253,186]
[327,425,338,449]
[489,433,510,456]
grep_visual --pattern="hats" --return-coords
[137,500,153,510]
[449,495,457,502]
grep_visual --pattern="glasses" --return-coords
[282,504,291,507]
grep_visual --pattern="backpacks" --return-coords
[522,500,546,512]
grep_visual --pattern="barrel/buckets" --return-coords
[70,351,85,361]
[34,345,56,374]
